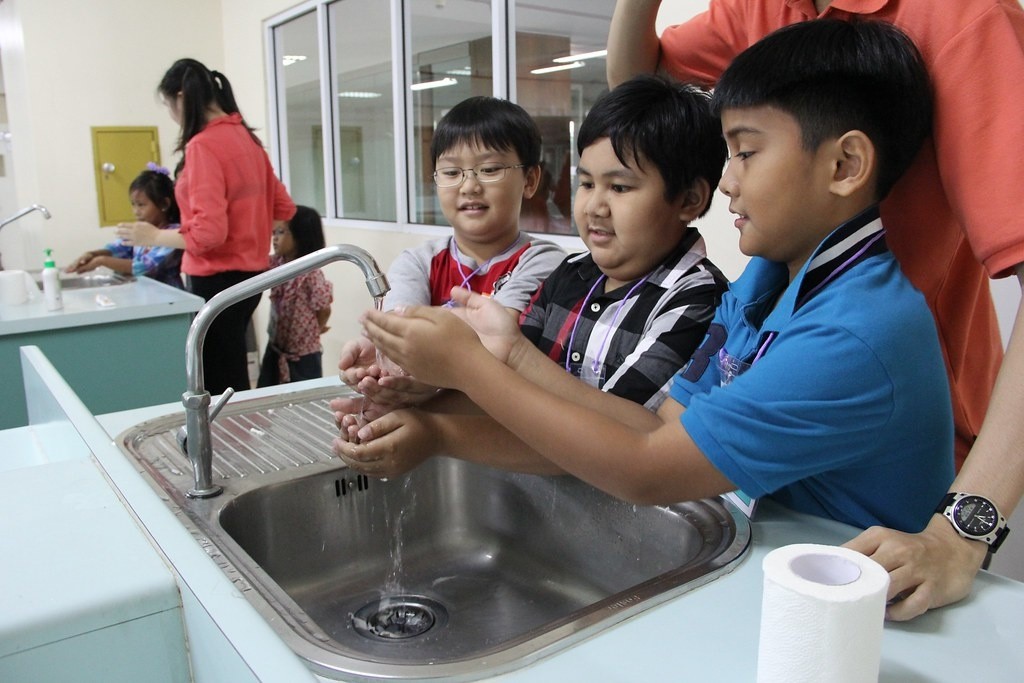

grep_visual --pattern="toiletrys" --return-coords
[42,248,64,311]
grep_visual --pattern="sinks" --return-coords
[36,271,139,293]
[217,454,755,683]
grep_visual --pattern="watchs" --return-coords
[934,491,1010,553]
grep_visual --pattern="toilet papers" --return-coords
[756,541,890,683]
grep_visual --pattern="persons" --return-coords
[332,16,957,533]
[607,0,1024,623]
[115,57,297,397]
[256,205,332,388]
[66,161,185,289]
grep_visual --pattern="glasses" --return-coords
[432,161,527,187]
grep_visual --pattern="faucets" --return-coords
[176,243,391,499]
[0,203,51,230]
